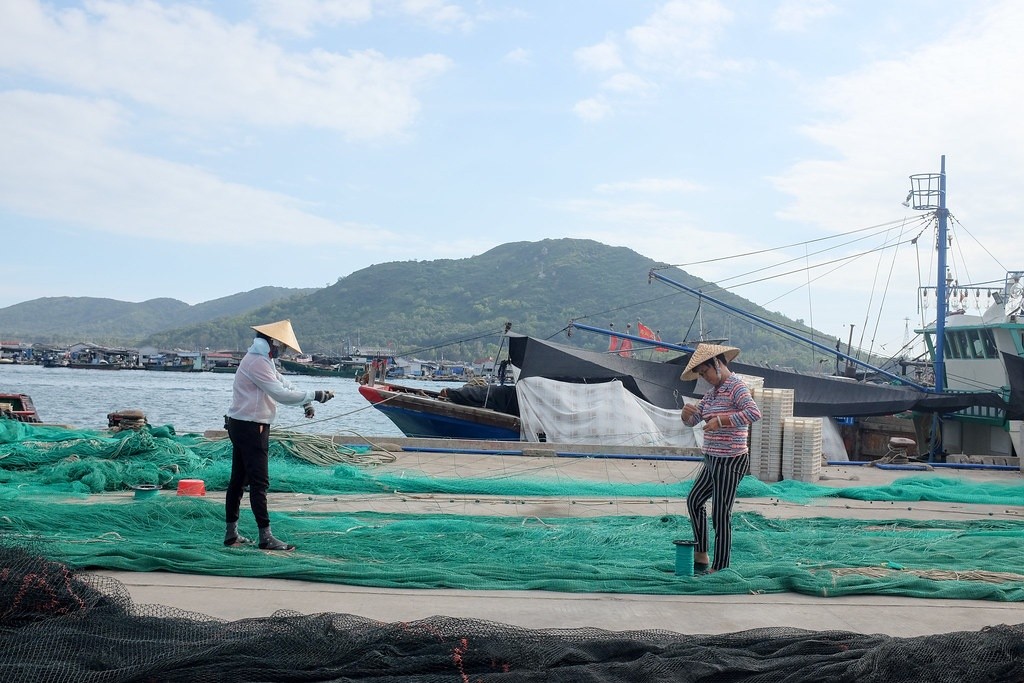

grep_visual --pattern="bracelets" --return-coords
[716,415,723,429]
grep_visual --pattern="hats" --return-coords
[250,318,303,355]
[679,343,741,382]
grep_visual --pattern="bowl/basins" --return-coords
[176,479,206,496]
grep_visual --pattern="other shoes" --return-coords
[695,561,708,572]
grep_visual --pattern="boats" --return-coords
[358,155,1024,464]
[0,342,509,382]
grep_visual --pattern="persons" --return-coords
[221,319,337,552]
[678,341,761,574]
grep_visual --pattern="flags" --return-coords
[608,322,670,358]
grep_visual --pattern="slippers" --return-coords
[258,543,295,552]
[224,541,256,547]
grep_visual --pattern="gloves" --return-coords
[303,402,315,419]
[315,390,335,404]
